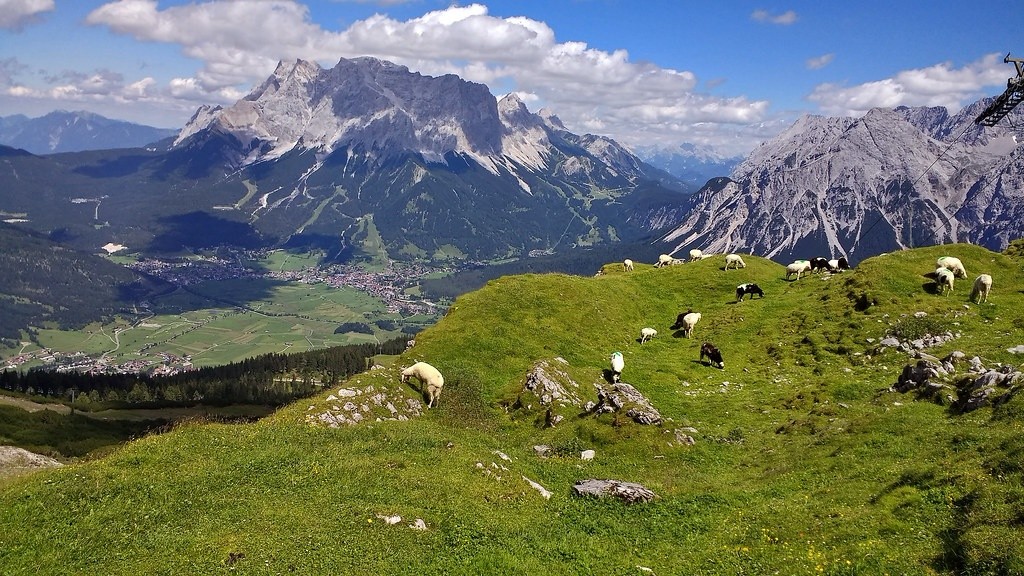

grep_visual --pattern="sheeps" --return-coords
[970,274,993,305]
[785,256,849,281]
[608,351,625,384]
[724,254,747,272]
[735,283,765,303]
[683,312,702,339]
[690,248,702,263]
[623,259,633,272]
[658,254,674,269]
[399,362,444,409]
[934,256,967,298]
[700,342,725,369]
[641,328,657,345]
